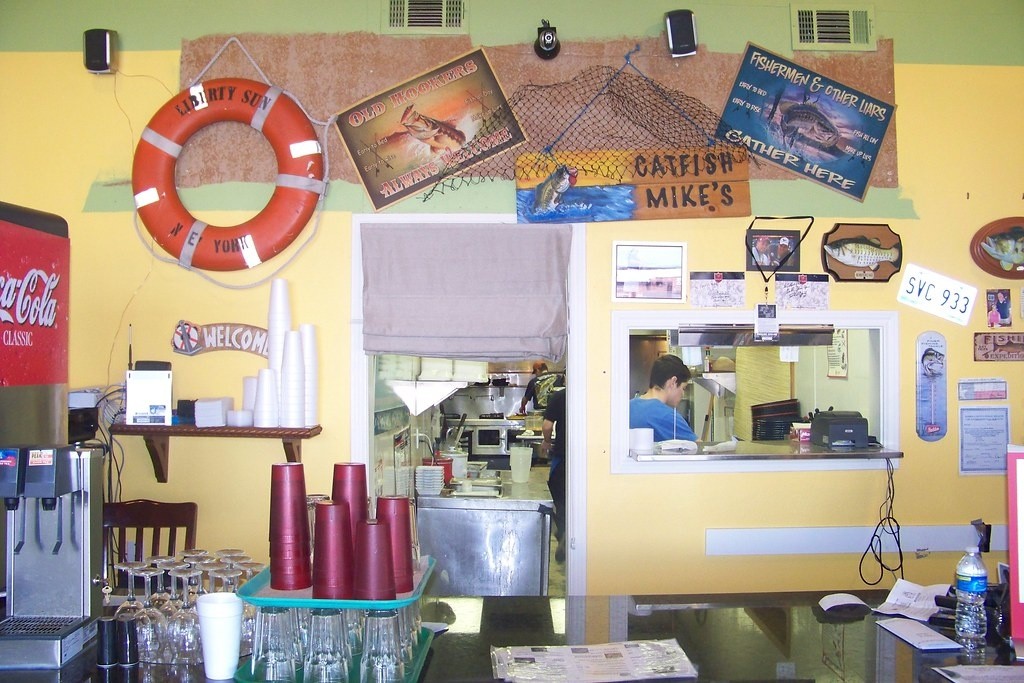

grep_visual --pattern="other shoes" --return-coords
[554,531,566,562]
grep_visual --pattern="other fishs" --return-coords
[993,341,1024,354]
[921,348,941,366]
[403,115,466,151]
[824,235,902,272]
[980,226,1024,271]
[533,166,578,215]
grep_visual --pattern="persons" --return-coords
[519,364,566,416]
[630,354,705,442]
[752,237,794,266]
[540,367,566,561]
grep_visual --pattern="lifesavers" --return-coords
[130,77,325,272]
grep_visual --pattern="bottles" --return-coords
[703,346,713,372]
[954,546,987,638]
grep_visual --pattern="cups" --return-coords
[267,461,414,601]
[509,446,533,483]
[252,606,425,683]
[241,278,320,429]
[440,448,469,480]
[635,427,653,449]
[196,592,243,680]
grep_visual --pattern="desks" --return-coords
[112,410,321,484]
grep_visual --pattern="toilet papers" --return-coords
[629,428,654,449]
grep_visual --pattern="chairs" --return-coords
[102,499,198,588]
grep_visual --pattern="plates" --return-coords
[414,465,445,496]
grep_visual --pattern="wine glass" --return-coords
[114,548,258,667]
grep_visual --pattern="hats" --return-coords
[531,360,547,374]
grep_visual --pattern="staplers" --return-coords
[929,595,1000,631]
